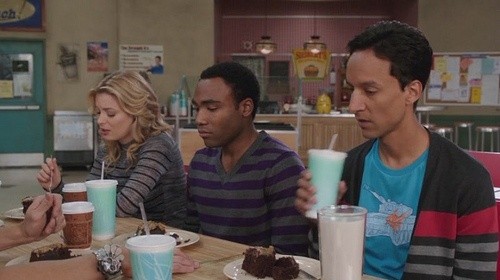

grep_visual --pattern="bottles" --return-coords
[179,74,191,116]
[316,92,331,114]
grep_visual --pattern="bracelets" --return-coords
[92,244,126,280]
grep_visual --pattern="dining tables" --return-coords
[0,207,391,280]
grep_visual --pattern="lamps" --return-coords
[304,0,327,53]
[255,0,278,54]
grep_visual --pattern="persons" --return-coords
[145,55,164,74]
[36,69,187,229]
[0,193,199,280]
[185,63,310,257]
[295,21,498,279]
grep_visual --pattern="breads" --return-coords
[22,195,36,214]
[241,245,299,280]
[29,243,72,263]
[135,221,166,235]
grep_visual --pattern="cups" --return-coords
[317,205,368,280]
[61,201,95,250]
[84,179,118,241]
[169,94,179,116]
[61,182,87,204]
[304,148,348,222]
[126,234,176,280]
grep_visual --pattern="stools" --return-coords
[424,120,500,153]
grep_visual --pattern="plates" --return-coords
[107,228,200,249]
[223,255,321,280]
[4,207,25,220]
[5,250,95,267]
